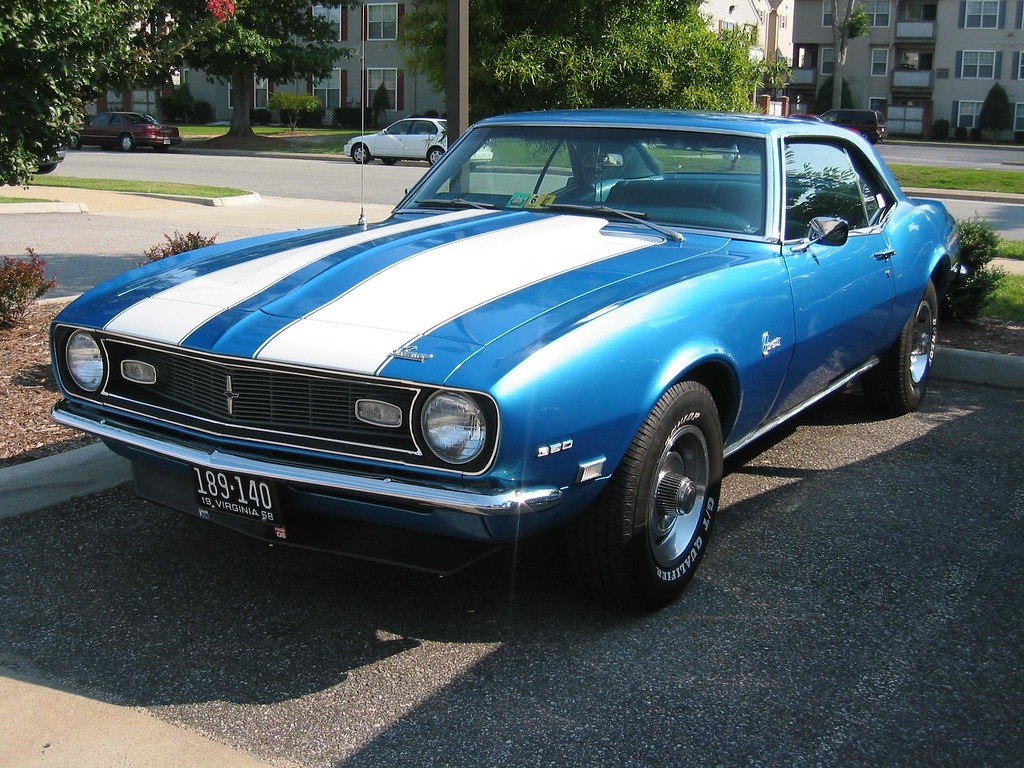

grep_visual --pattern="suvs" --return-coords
[820,108,888,145]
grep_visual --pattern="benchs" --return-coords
[605,181,749,217]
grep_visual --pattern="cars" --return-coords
[344,117,494,172]
[788,114,868,145]
[66,113,183,151]
[47,113,960,608]
